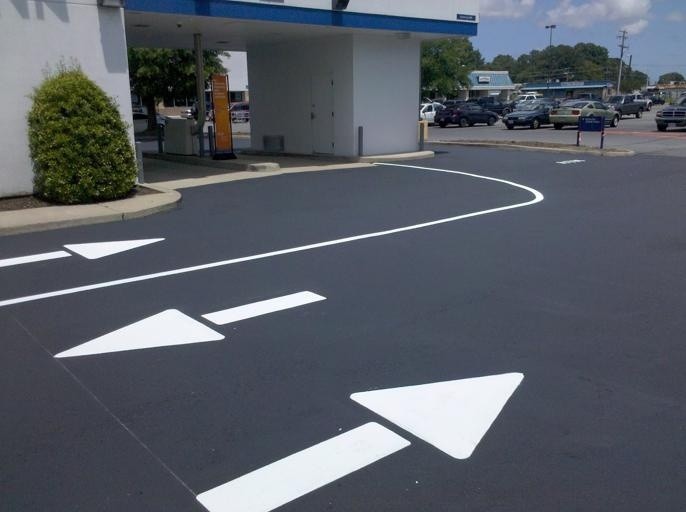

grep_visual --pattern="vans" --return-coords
[208,100,251,123]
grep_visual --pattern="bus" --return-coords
[130,86,147,134]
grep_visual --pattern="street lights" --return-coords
[545,24,556,90]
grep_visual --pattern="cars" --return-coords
[155,111,168,128]
[419,92,686,129]
[180,109,195,119]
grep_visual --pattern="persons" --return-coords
[509,101,516,112]
